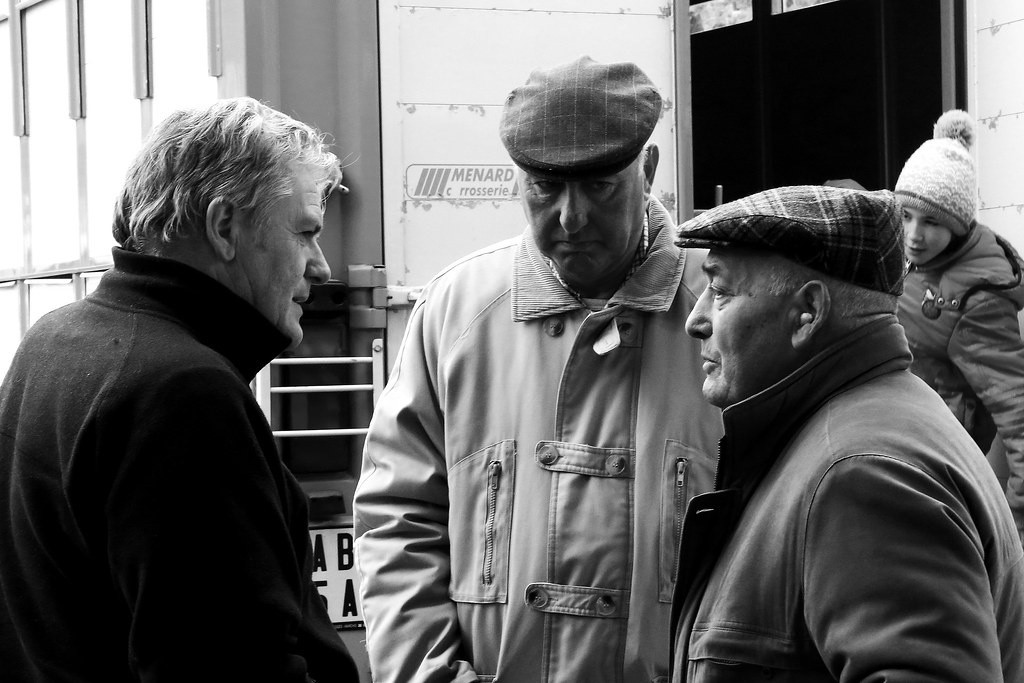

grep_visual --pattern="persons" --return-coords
[894,108,1024,549]
[1,99,361,683]
[355,51,722,683]
[662,186,1024,683]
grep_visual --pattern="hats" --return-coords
[673,185,905,296]
[894,108,979,237]
[500,55,661,178]
